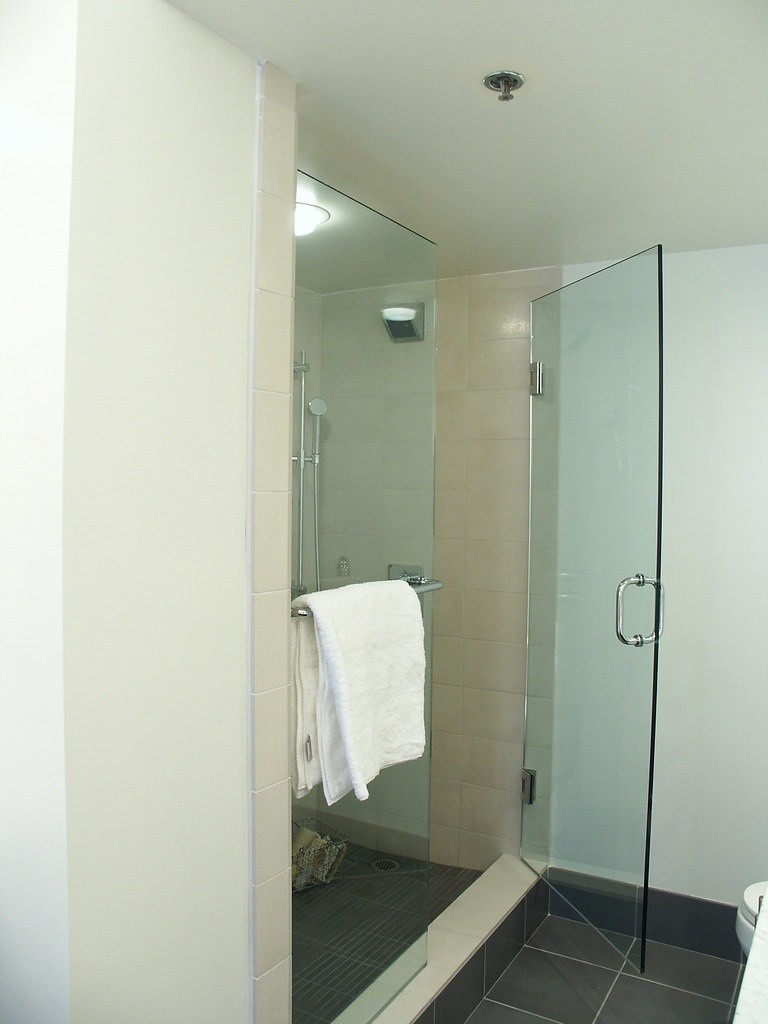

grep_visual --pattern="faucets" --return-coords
[308,397,328,454]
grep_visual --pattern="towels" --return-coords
[291,574,431,809]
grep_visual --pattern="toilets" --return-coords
[733,880,768,961]
